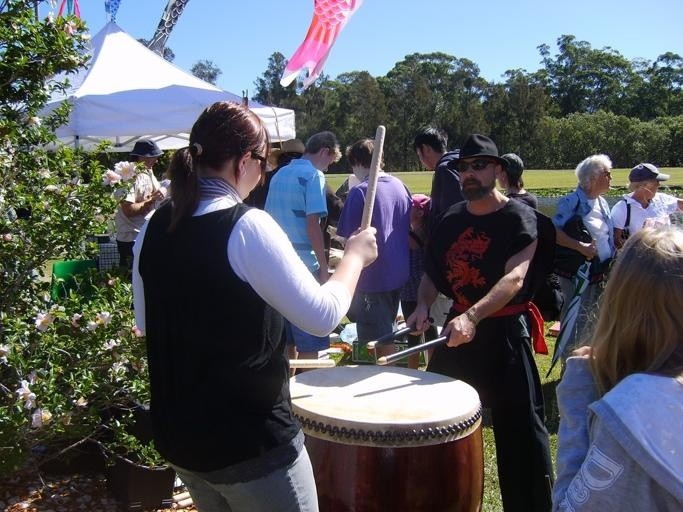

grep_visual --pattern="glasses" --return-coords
[246,148,268,169]
[602,170,611,177]
[453,158,497,172]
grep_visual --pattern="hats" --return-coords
[410,192,430,209]
[446,133,511,171]
[129,138,165,158]
[268,139,306,170]
[628,162,671,183]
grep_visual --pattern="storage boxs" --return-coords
[352,336,428,365]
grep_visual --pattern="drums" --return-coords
[287,366,484,512]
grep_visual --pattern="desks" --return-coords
[98,243,121,274]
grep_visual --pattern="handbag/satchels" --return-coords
[608,198,631,245]
[552,192,591,277]
